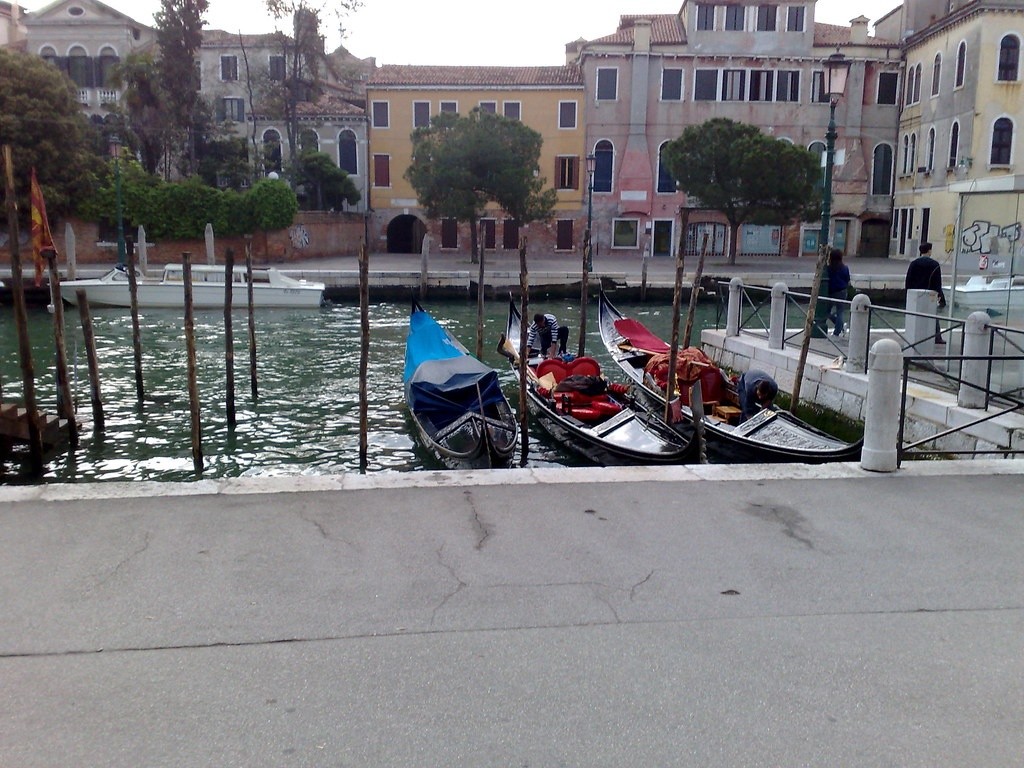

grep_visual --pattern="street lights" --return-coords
[584,149,598,272]
[809,44,851,339]
[108,135,127,262]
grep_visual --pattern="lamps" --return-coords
[958,155,973,176]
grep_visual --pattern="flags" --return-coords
[30,167,57,286]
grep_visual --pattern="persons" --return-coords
[526,313,569,359]
[905,243,948,344]
[737,369,778,424]
[828,248,851,336]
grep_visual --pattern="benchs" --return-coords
[536,356,608,406]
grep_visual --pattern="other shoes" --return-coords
[828,333,840,343]
[935,339,947,344]
[843,329,851,340]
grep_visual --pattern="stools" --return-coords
[717,406,742,424]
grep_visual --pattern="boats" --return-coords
[47,260,329,309]
[594,274,865,462]
[496,289,710,466]
[403,284,520,469]
